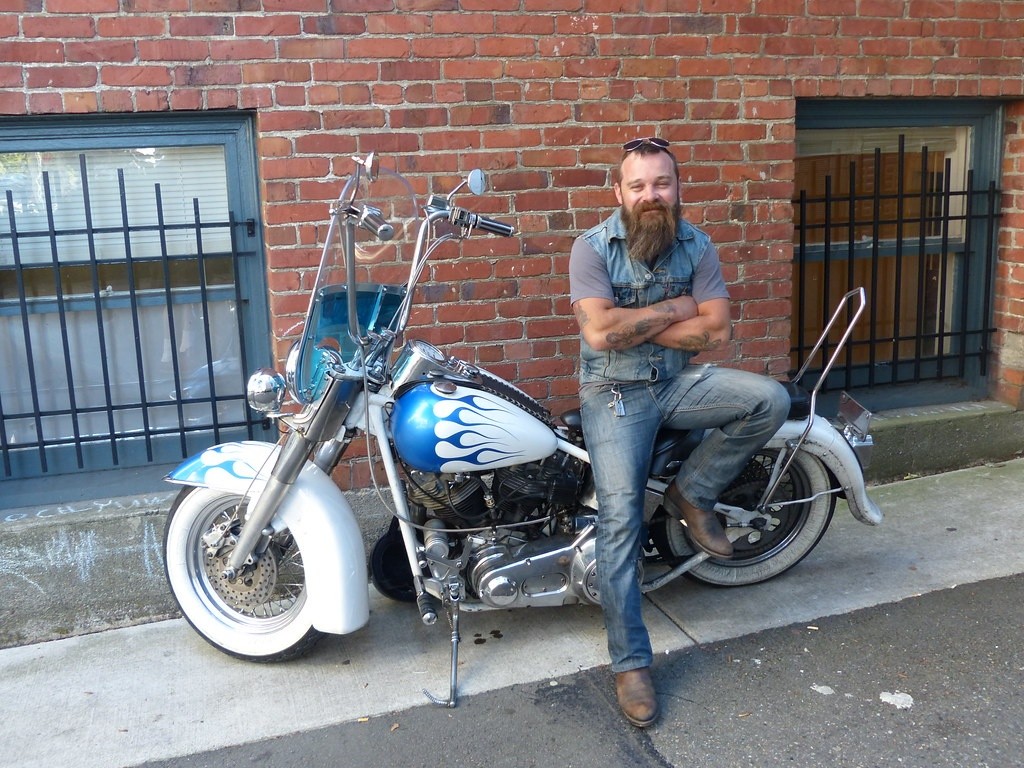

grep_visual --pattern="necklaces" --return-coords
[608,393,625,420]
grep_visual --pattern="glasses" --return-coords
[621,138,672,159]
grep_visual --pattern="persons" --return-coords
[569,143,791,728]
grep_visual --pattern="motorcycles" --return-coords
[162,150,882,708]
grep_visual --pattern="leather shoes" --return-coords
[616,665,660,727]
[663,478,734,560]
